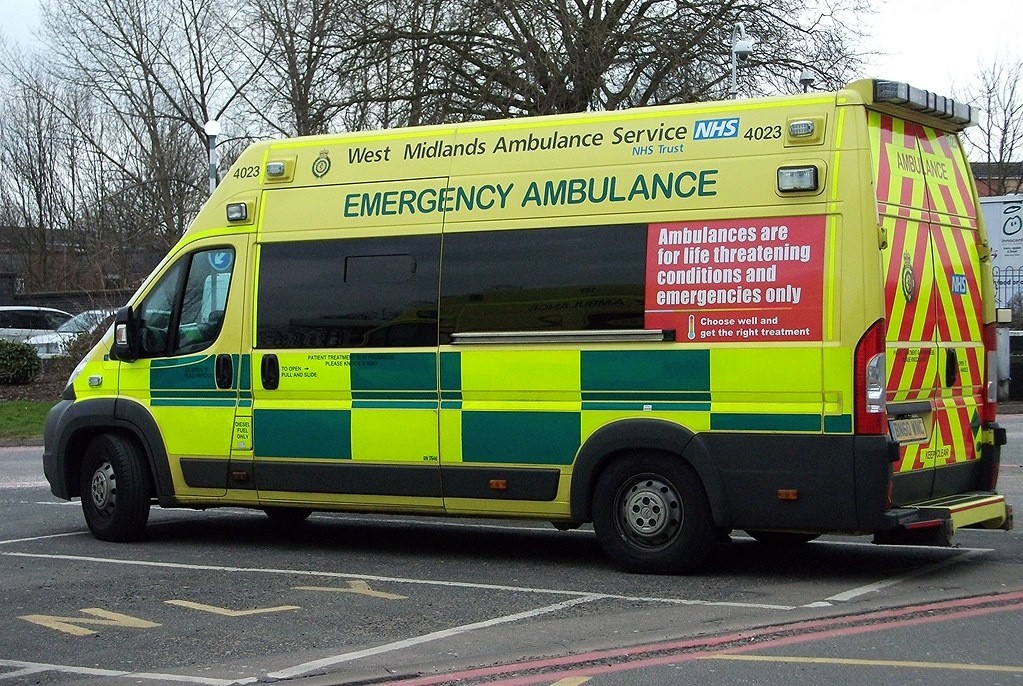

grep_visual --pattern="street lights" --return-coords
[728,22,755,99]
[799,70,815,93]
[202,119,221,313]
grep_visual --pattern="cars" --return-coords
[0,305,90,348]
[22,307,171,362]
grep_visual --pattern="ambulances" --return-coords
[37,78,1015,578]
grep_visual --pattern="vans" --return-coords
[200,271,231,323]
